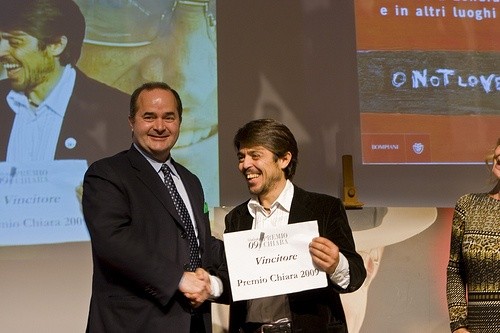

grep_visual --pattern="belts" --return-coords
[192,313,202,318]
[243,320,292,333]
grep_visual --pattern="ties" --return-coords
[160,164,203,270]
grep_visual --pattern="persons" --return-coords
[446,137,500,333]
[191,118,367,333]
[82,81,225,333]
[0,0,143,243]
